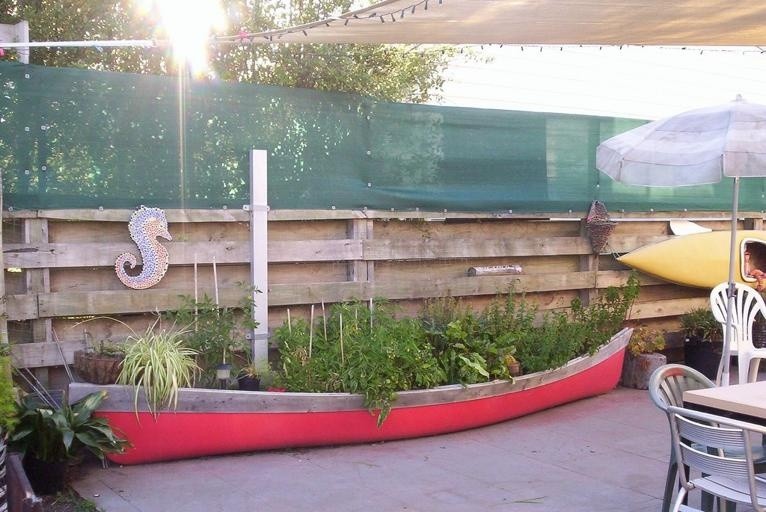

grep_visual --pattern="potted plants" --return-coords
[485,329,520,374]
[5,279,290,471]
[627,326,667,358]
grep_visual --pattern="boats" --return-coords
[67,323,634,466]
[616,229,766,292]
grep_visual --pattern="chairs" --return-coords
[647,281,766,512]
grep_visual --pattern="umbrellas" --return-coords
[594,94,766,387]
